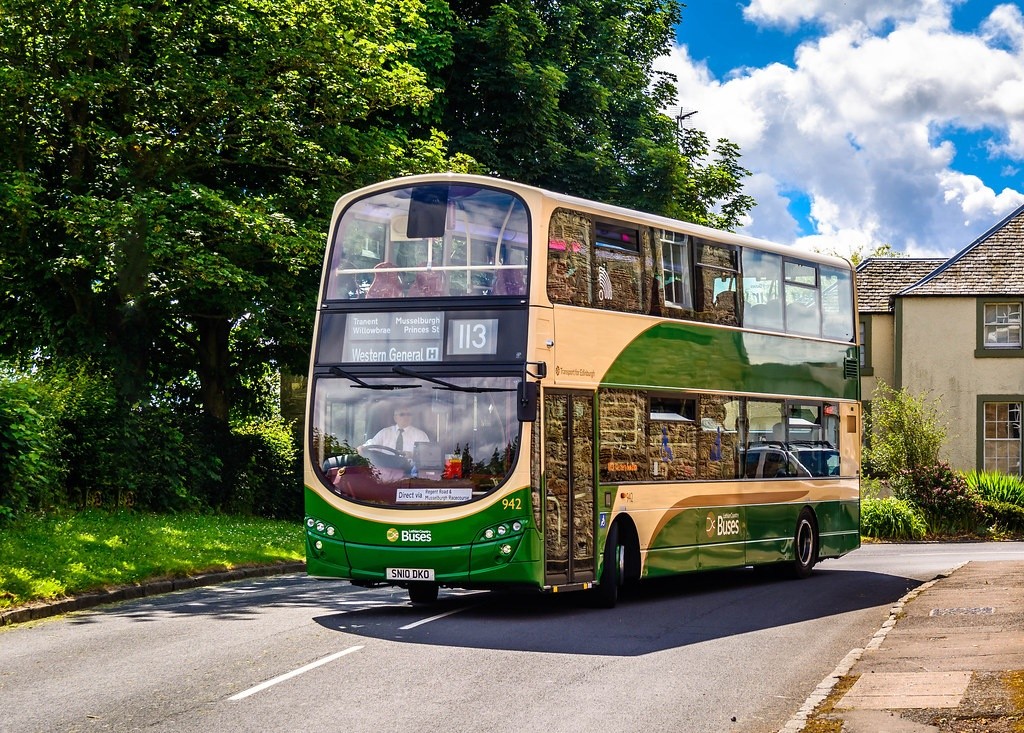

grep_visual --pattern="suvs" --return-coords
[740,440,840,479]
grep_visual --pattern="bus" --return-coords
[302,173,862,609]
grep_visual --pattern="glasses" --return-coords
[766,459,779,463]
[395,412,412,417]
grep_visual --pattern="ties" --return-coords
[396,429,404,453]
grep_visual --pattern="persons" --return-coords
[357,408,430,466]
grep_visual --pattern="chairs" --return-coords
[364,261,405,299]
[715,292,826,335]
[406,261,451,297]
[490,264,527,295]
